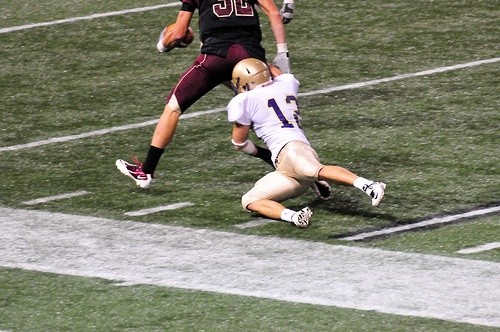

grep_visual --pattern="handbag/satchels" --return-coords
[201,36,233,57]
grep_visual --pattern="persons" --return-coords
[116,0,291,188]
[226,58,387,228]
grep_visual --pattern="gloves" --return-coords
[280,0,294,24]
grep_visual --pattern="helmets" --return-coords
[233,58,271,94]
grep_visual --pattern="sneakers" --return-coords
[290,207,313,228]
[311,179,332,200]
[363,181,387,207]
[116,157,152,190]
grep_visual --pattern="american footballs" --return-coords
[163,23,195,46]
